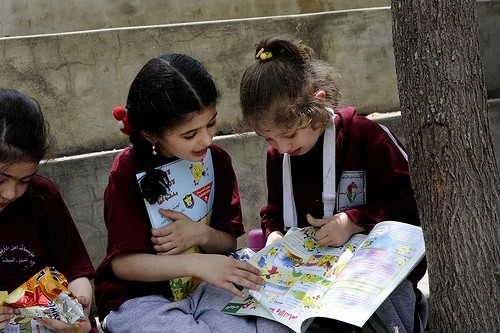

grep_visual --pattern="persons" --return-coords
[0,87,96,333]
[239,33,427,333]
[93,53,292,333]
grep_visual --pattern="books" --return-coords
[135,146,215,302]
[220,220,426,333]
